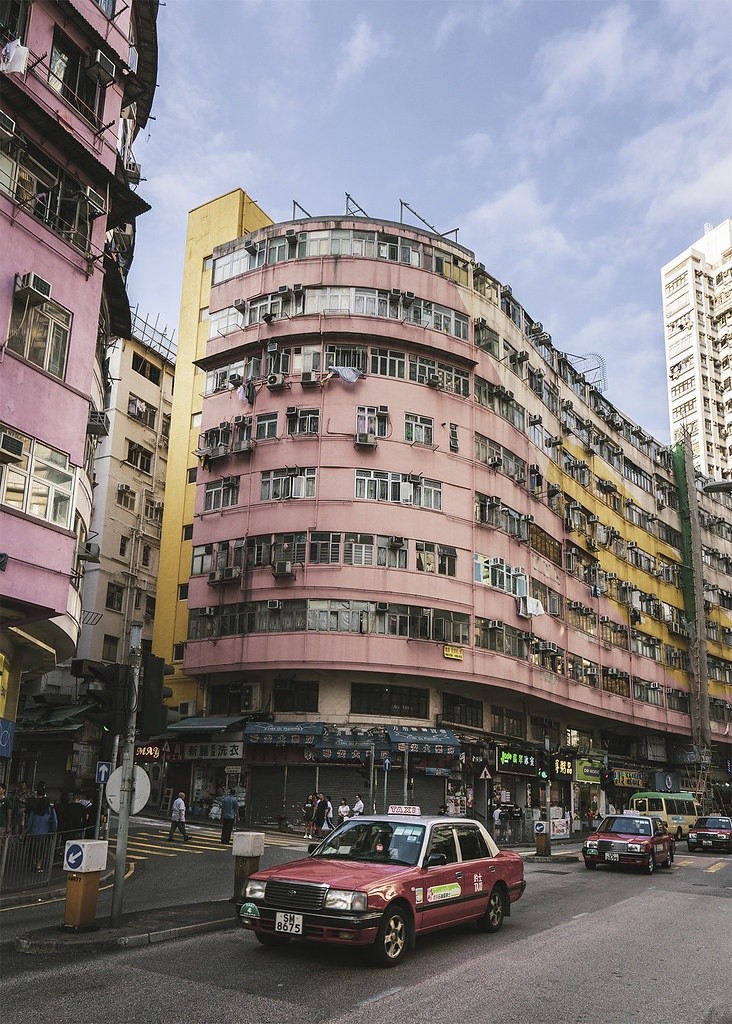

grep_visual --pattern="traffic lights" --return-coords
[600,770,611,789]
[539,750,549,780]
[408,755,422,777]
[84,662,129,736]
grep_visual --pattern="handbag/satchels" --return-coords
[48,807,55,833]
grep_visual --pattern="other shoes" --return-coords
[493,837,522,845]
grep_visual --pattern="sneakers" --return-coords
[315,835,320,841]
[303,835,308,839]
[308,834,312,840]
[320,836,324,841]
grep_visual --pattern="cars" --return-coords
[582,810,677,875]
[686,813,732,854]
[234,805,527,971]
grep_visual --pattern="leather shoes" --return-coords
[184,836,192,841]
[168,837,174,841]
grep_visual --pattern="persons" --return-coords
[0,779,732,873]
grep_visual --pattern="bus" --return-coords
[628,792,700,840]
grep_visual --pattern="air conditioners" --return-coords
[90,410,112,436]
[154,502,165,510]
[0,431,26,463]
[113,221,135,249]
[193,230,732,711]
[1,108,17,143]
[21,271,53,302]
[85,47,116,87]
[76,542,101,561]
[124,161,142,187]
[74,184,107,216]
[118,483,131,493]
